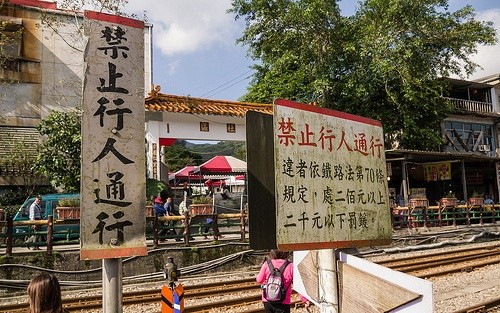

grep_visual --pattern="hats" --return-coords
[154,198,163,203]
[37,195,42,199]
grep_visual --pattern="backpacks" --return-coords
[261,260,292,304]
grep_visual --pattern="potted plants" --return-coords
[409,193,429,208]
[469,192,485,205]
[441,193,457,207]
[56,196,81,218]
[189,196,213,215]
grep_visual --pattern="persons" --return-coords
[165,196,177,241]
[255,248,310,313]
[23,194,45,250]
[152,196,167,242]
[175,196,195,242]
[220,186,232,200]
[203,217,225,239]
[27,273,69,313]
[483,193,495,204]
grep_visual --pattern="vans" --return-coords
[13,193,80,239]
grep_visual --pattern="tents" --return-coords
[169,155,246,194]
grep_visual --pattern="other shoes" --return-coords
[33,247,42,250]
[24,242,29,250]
[175,239,183,241]
[190,238,195,240]
[219,235,225,238]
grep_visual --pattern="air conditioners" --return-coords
[478,145,490,152]
